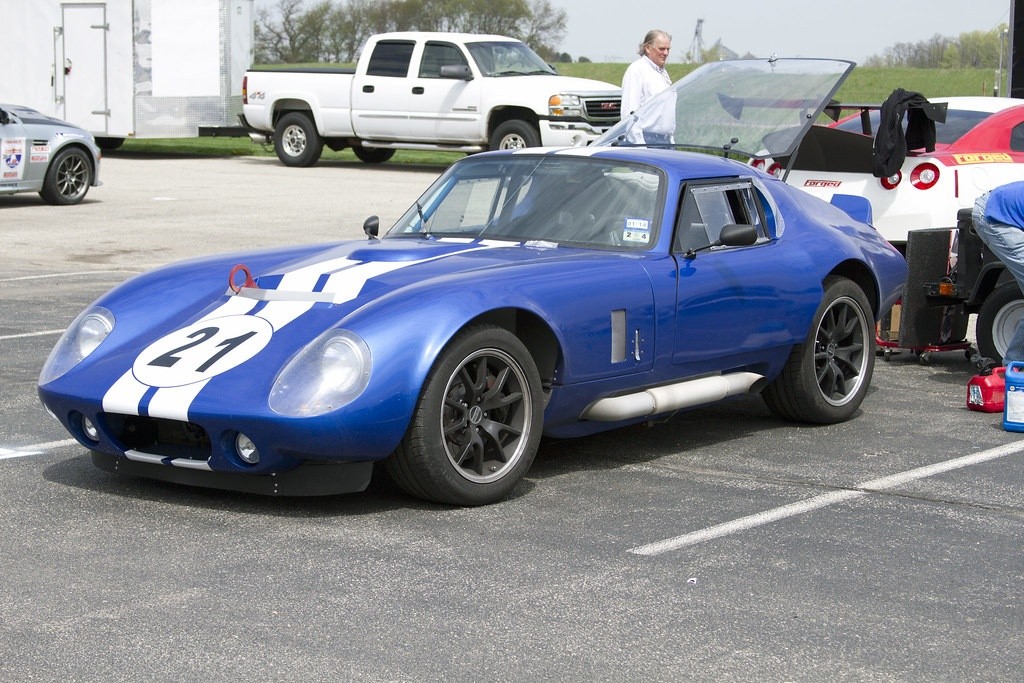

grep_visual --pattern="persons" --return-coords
[972,180,1024,371]
[620,30,677,149]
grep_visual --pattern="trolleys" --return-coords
[875,299,977,366]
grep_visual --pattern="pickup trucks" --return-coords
[238,31,623,165]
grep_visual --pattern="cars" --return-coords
[0,103,104,206]
[744,96,1024,246]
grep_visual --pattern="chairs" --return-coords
[495,175,608,243]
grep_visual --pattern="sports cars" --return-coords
[34,55,911,508]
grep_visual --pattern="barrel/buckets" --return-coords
[1003,361,1024,433]
[967,364,1018,413]
